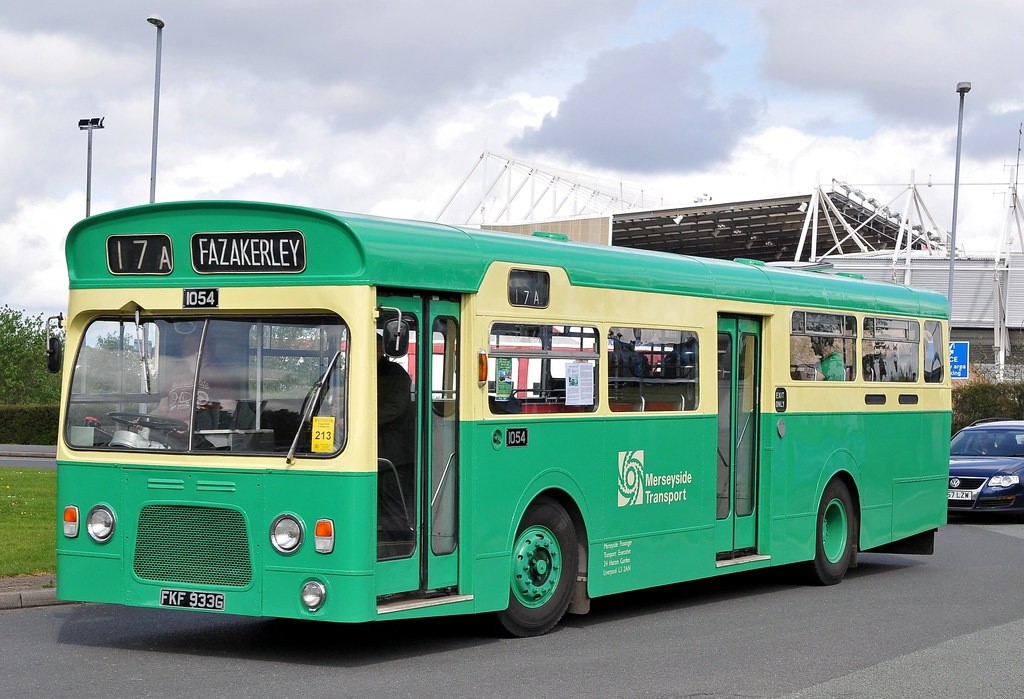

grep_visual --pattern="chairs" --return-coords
[791,362,876,382]
[512,390,687,414]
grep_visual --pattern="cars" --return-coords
[949,417,1024,523]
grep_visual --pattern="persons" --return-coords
[862,335,910,384]
[132,329,237,435]
[807,325,845,381]
[978,435,996,454]
[334,333,416,542]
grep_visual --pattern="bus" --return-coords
[44,201,955,642]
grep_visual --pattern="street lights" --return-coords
[145,17,166,203]
[947,80,972,341]
[77,116,105,219]
